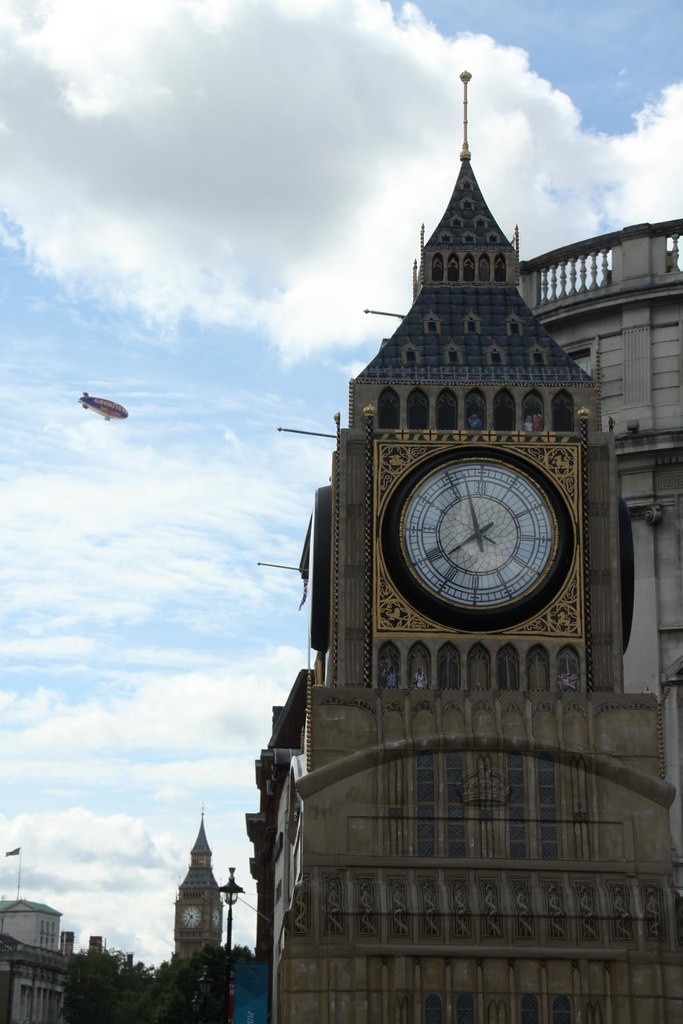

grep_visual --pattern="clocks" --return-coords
[182,906,201,928]
[212,908,221,929]
[400,457,560,610]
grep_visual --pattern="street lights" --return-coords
[217,865,246,1024]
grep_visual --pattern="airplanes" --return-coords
[79,391,129,423]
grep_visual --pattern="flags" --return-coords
[5,847,20,856]
[233,960,270,1024]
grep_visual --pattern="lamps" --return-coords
[627,419,639,432]
